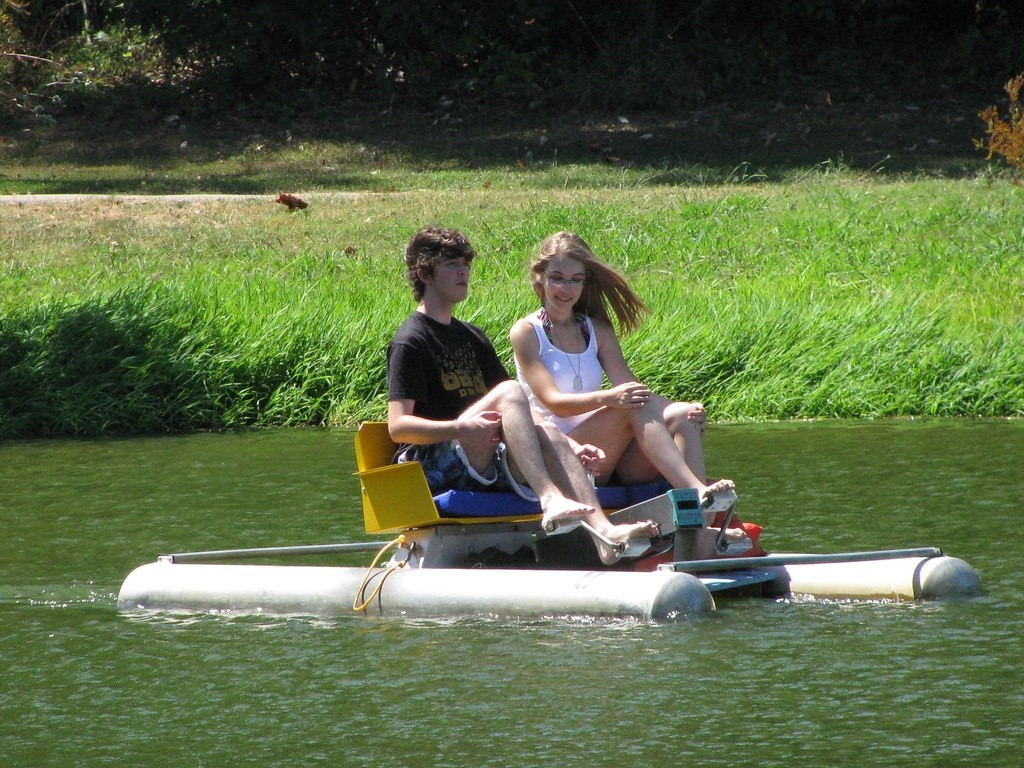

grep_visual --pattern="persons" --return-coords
[386,223,662,566]
[509,230,748,561]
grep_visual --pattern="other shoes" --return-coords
[464,544,538,570]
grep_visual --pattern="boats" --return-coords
[118,417,983,622]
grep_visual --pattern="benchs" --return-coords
[353,421,673,535]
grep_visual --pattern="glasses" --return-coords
[543,270,590,286]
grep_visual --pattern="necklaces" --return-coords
[549,315,584,392]
